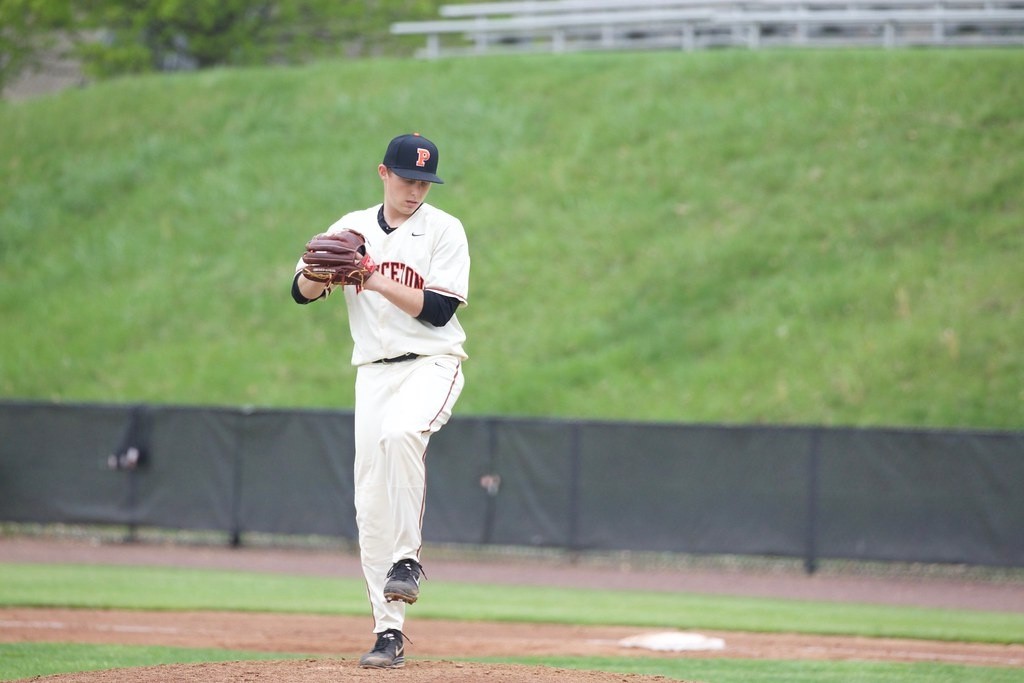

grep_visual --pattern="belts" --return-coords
[372,352,418,363]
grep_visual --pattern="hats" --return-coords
[383,132,445,185]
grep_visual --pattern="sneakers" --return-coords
[359,630,406,669]
[384,560,421,604]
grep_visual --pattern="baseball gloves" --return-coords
[302,229,378,285]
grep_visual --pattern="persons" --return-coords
[290,133,472,669]
